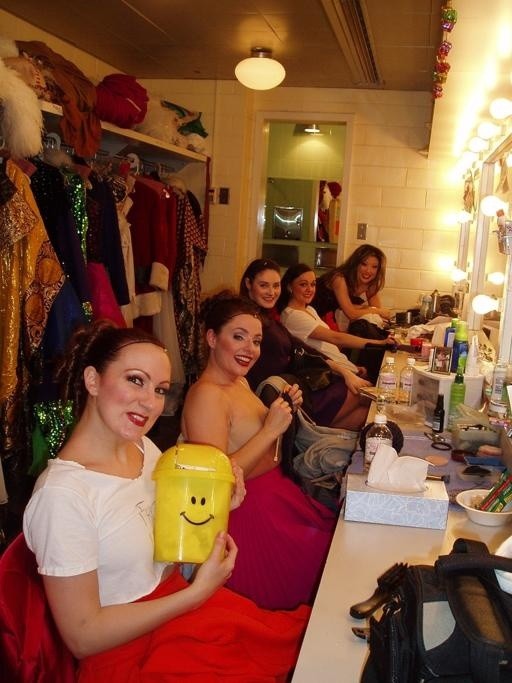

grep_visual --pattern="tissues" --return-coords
[342,443,450,530]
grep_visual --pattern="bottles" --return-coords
[399,358,416,414]
[444,317,470,372]
[431,394,446,432]
[455,289,464,309]
[432,289,440,313]
[364,414,393,470]
[450,367,466,414]
[420,296,432,323]
[377,357,396,412]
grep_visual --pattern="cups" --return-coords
[411,334,424,358]
[422,342,432,361]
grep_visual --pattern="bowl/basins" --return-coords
[456,487,512,524]
[396,313,412,325]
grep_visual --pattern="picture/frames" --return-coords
[430,345,453,372]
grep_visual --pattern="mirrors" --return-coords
[461,177,478,276]
[478,134,512,355]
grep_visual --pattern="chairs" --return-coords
[0,531,79,683]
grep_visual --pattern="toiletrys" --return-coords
[465,335,480,377]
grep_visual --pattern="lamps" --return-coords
[304,124,321,134]
[233,47,287,92]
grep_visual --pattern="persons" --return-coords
[274,264,400,377]
[23,319,312,682]
[310,243,397,333]
[175,288,338,611]
[240,258,372,433]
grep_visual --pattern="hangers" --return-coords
[0,132,176,177]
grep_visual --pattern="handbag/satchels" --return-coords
[359,537,511,683]
[292,346,336,392]
[340,320,385,381]
[334,306,384,330]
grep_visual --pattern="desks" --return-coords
[291,320,512,683]
